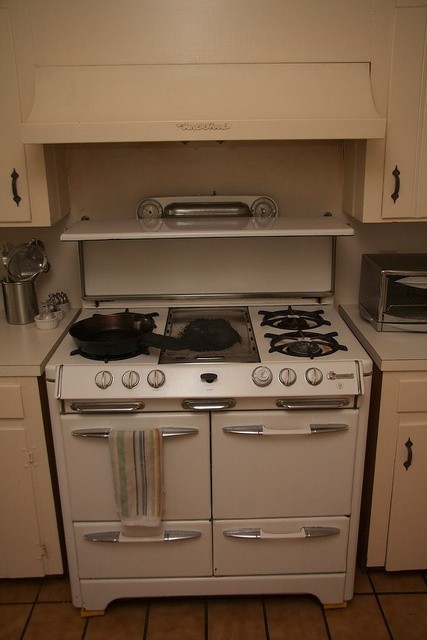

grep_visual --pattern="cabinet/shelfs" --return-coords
[0,0,68,226]
[361,369,427,572]
[1,374,64,577]
[345,0,426,224]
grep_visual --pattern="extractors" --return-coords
[20,63,388,145]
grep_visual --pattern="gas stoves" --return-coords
[46,307,373,369]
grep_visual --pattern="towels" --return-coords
[108,425,163,525]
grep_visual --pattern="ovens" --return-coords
[47,398,369,611]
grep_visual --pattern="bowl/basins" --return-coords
[52,309,64,321]
[57,301,71,311]
[34,314,58,329]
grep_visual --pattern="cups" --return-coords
[0,277,39,324]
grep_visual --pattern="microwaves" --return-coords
[357,252,427,331]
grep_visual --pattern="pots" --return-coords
[68,312,189,359]
